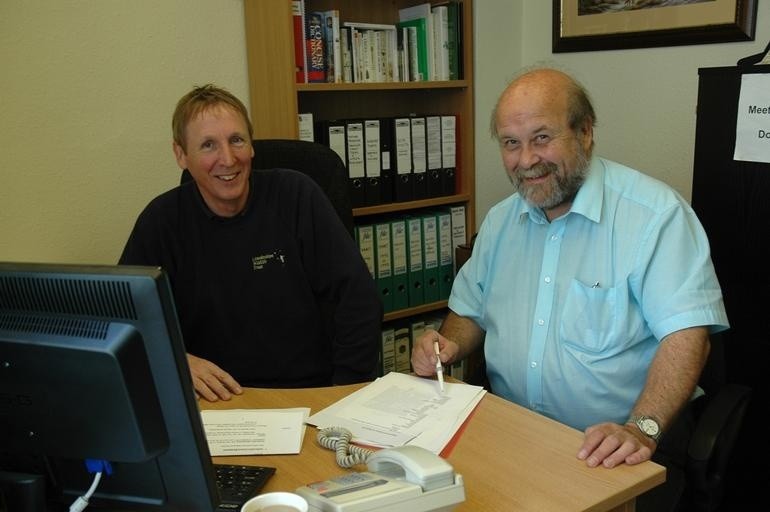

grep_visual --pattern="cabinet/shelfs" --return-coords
[242,0,476,379]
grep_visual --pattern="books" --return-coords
[293,1,463,87]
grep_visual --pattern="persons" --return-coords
[115,86,383,403]
[409,68,730,469]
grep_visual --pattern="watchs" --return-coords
[625,414,664,444]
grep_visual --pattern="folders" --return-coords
[375,314,463,381]
[299,113,457,207]
[351,206,467,316]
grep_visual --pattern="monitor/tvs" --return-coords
[0,261,220,512]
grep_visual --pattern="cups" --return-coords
[240,492,309,512]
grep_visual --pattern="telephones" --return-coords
[296,445,466,512]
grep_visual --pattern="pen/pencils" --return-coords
[433,339,444,392]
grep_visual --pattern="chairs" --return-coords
[183,140,384,379]
[660,327,751,512]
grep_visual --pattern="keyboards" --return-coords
[213,464,277,512]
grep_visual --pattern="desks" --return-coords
[167,370,667,512]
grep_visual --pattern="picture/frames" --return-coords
[552,0,760,54]
